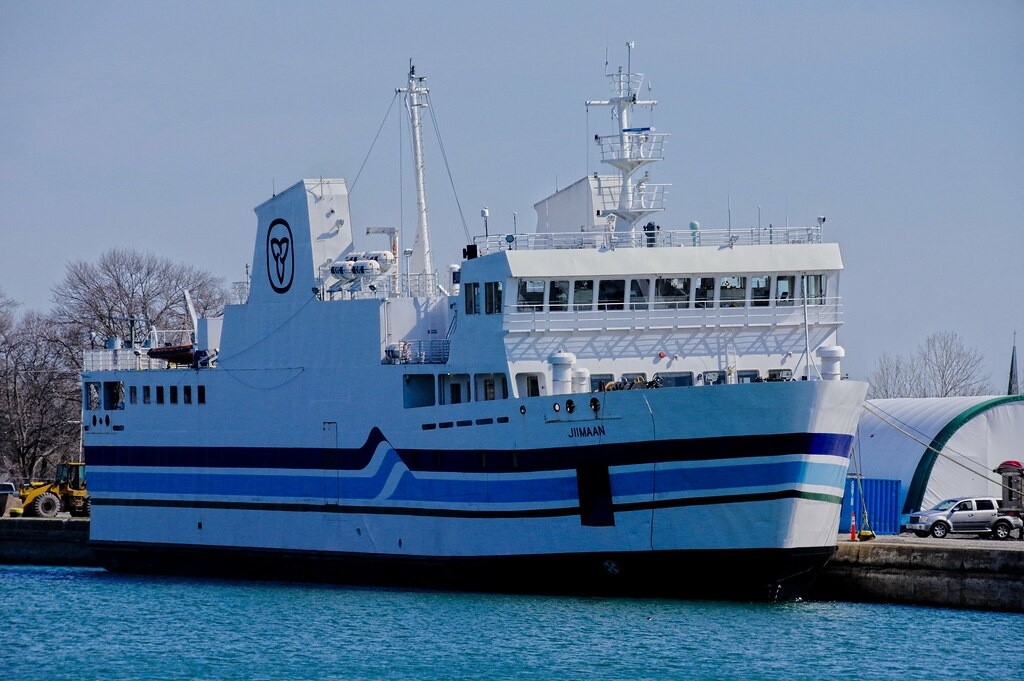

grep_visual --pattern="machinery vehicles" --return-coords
[0,463,91,517]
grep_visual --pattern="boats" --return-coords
[80,40,870,600]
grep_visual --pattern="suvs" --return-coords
[906,498,1023,540]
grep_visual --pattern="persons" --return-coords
[67,469,75,487]
